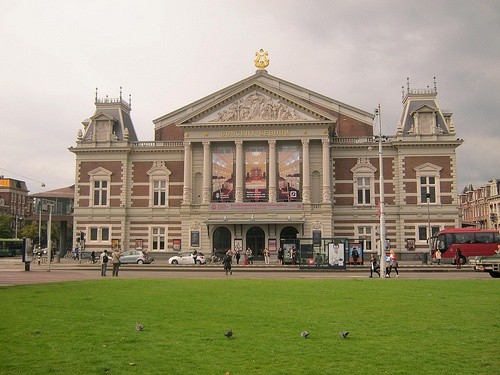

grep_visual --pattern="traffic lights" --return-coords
[75,232,80,243]
[80,232,84,241]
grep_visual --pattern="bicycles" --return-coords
[205,255,222,265]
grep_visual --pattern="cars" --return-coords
[475,248,500,278]
[168,252,206,265]
[119,250,151,264]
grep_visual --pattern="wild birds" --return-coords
[341,331,350,338]
[301,331,310,339]
[224,330,234,338]
[135,323,144,330]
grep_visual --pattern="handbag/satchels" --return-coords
[103,256,109,263]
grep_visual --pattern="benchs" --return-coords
[300,263,321,268]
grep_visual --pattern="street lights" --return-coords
[15,215,22,239]
[367,102,391,280]
[425,192,434,264]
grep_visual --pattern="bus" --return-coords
[426,229,500,265]
[0,238,23,257]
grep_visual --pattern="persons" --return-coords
[264,248,270,264]
[455,247,462,268]
[292,245,298,264]
[246,247,254,264]
[494,244,500,254]
[435,249,442,264]
[37,246,45,261]
[227,249,233,264]
[234,249,241,264]
[314,252,322,267]
[330,243,342,264]
[278,247,284,265]
[100,249,109,276]
[369,253,380,277]
[112,249,121,276]
[193,248,197,264]
[352,247,359,267]
[73,246,79,260]
[119,248,123,257]
[211,248,217,262]
[386,249,399,277]
[222,252,231,275]
[90,250,95,264]
